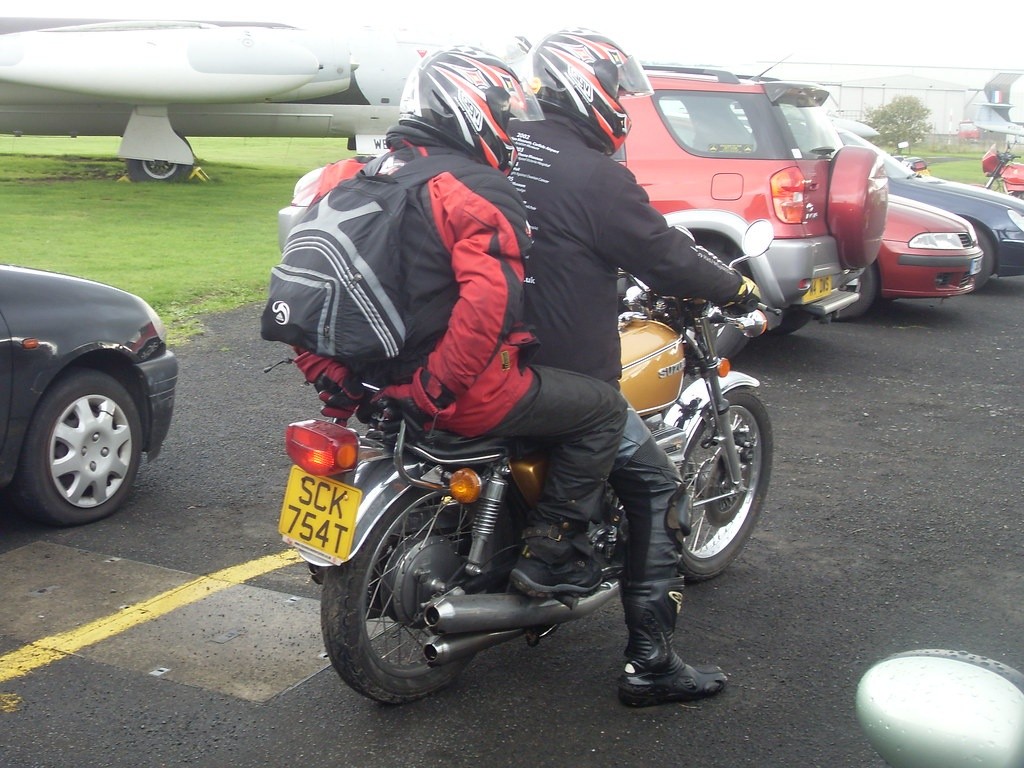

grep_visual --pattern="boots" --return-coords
[617,574,728,707]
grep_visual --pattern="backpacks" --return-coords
[260,148,478,370]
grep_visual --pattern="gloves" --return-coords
[720,268,762,318]
[292,350,365,426]
[364,365,456,440]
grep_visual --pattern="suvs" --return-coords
[278,63,889,364]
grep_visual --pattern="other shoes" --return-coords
[511,544,602,598]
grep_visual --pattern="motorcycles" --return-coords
[279,215,809,707]
[972,138,1024,198]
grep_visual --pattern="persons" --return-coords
[293,46,628,600]
[506,28,762,707]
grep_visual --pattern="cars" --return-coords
[0,268,180,529]
[810,193,986,325]
[735,110,1024,276]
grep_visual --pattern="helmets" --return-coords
[523,26,655,156]
[398,46,546,178]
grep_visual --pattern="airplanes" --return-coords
[1,2,878,183]
